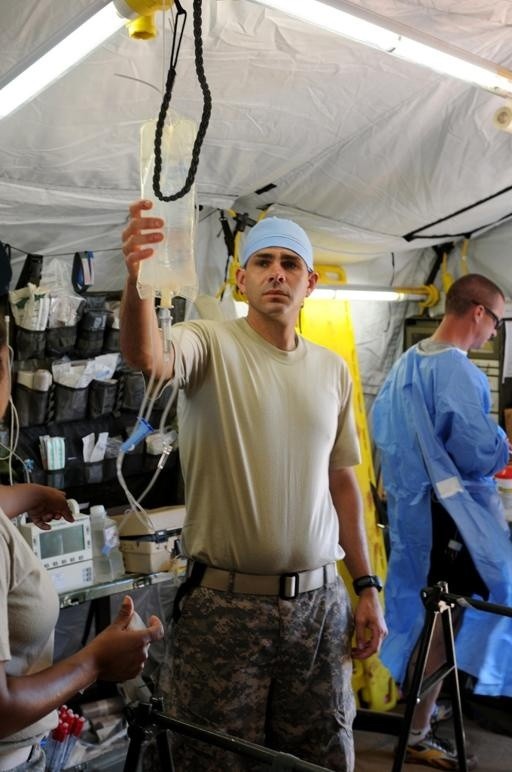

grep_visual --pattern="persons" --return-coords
[119,198,388,772]
[0,315,168,772]
[369,275,512,770]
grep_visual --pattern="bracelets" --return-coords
[353,574,382,596]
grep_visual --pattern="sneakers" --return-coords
[405,733,479,772]
[430,700,453,724]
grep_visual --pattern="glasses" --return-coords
[471,297,505,331]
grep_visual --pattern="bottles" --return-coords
[88,504,125,582]
[493,465,512,523]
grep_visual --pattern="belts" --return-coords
[184,556,339,604]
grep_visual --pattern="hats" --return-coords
[237,216,315,275]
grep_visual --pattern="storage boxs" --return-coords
[114,503,186,577]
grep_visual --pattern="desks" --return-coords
[51,560,182,693]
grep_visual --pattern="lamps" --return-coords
[0,1,183,126]
[241,0,511,100]
